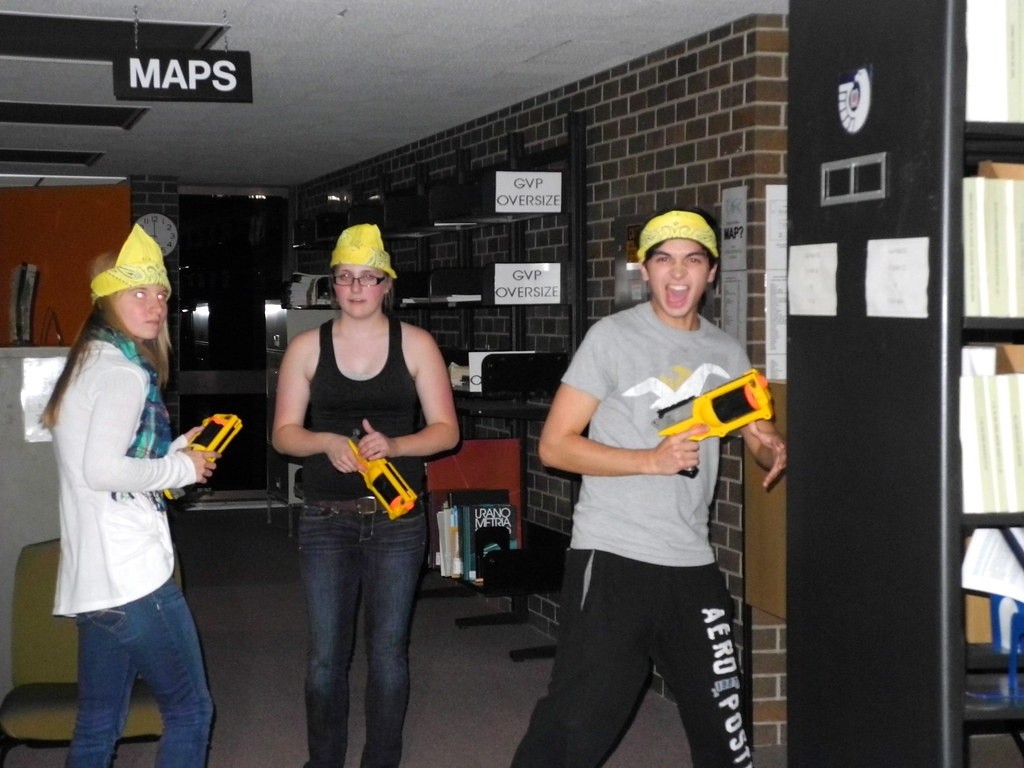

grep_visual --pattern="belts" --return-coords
[303,491,424,514]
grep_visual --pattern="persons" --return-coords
[51,249,222,768]
[511,208,787,768]
[271,224,461,768]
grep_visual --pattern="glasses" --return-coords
[332,275,385,286]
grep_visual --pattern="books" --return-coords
[957,342,1023,514]
[964,0,1024,124]
[961,148,1024,320]
[435,488,517,581]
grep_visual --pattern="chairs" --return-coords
[0,538,182,741]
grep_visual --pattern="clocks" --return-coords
[135,212,179,257]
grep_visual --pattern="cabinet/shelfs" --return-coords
[282,111,588,662]
[786,0,1024,768]
